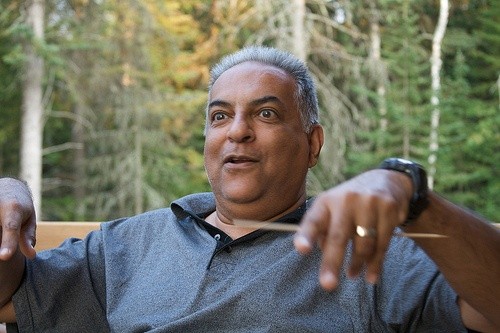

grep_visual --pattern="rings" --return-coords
[356,224,378,238]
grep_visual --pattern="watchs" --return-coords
[380,156,430,228]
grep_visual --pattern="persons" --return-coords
[0,46,500,333]
